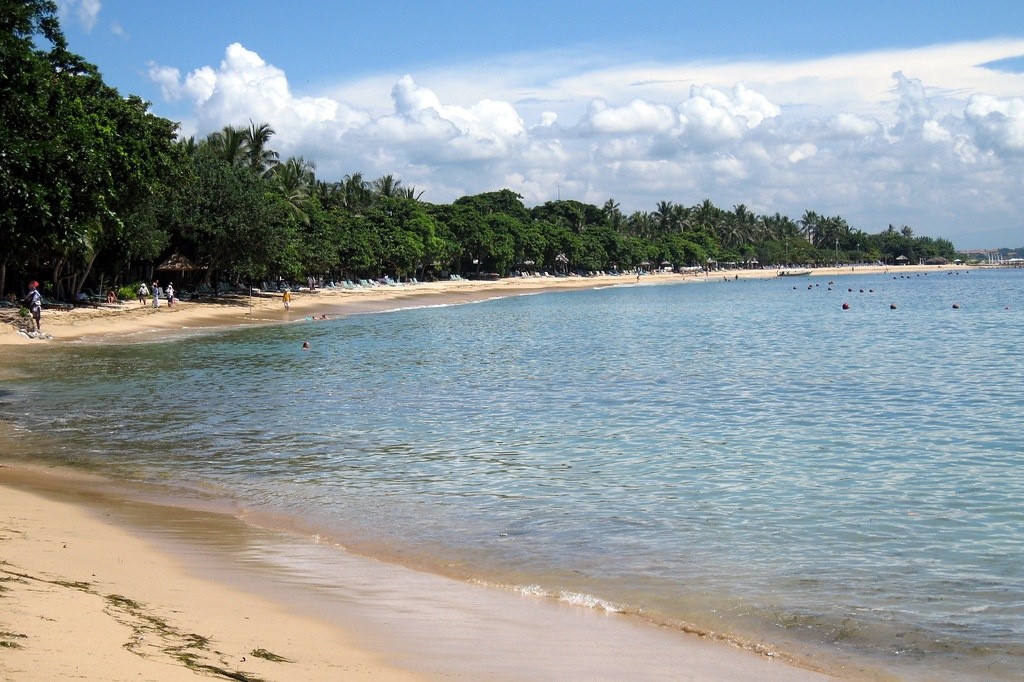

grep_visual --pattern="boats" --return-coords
[776,271,812,276]
[41,302,74,309]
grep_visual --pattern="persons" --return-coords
[152,278,160,308]
[138,283,150,305]
[107,289,117,304]
[306,276,317,291]
[166,280,174,308]
[79,290,90,302]
[23,281,42,331]
[283,289,292,310]
[302,340,309,350]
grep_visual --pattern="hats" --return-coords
[170,282,173,284]
[156,280,159,282]
[141,283,146,286]
[284,289,288,291]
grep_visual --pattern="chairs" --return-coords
[237,274,425,294]
[450,275,461,282]
[624,269,673,276]
[524,272,531,279]
[680,266,742,275]
[544,272,555,278]
[537,272,542,278]
[534,272,538,278]
[569,270,622,278]
[456,275,469,282]
[521,272,527,280]
